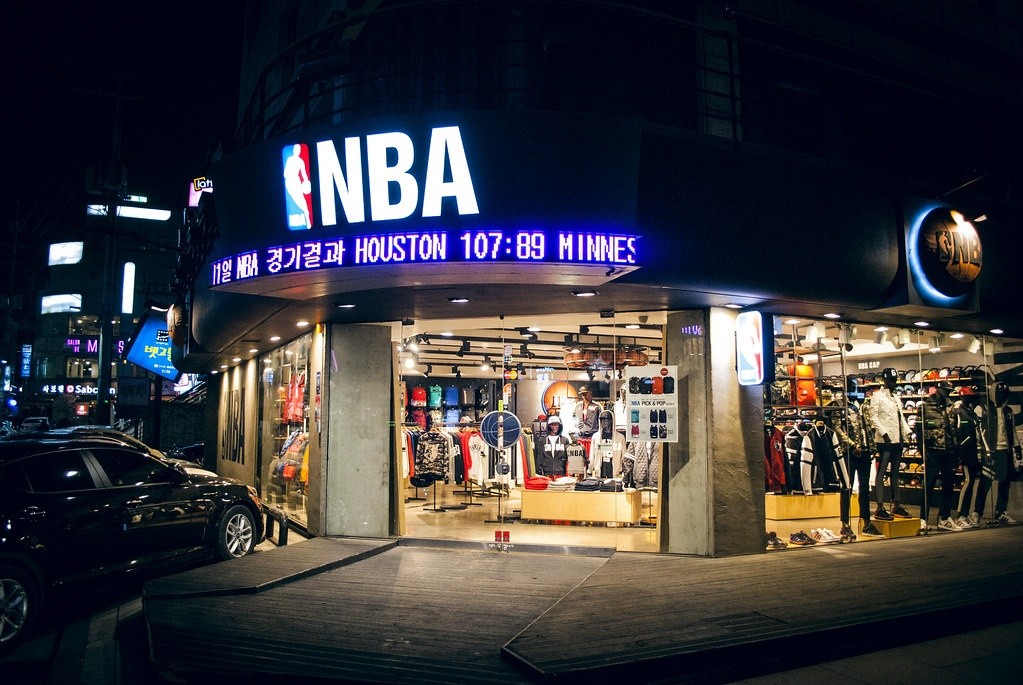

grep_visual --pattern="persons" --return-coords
[831,375,884,538]
[38,420,49,431]
[971,383,1023,526]
[917,381,963,532]
[870,368,913,519]
[57,412,72,428]
[576,385,602,438]
[563,429,587,477]
[954,386,991,528]
[587,410,626,479]
[536,416,568,477]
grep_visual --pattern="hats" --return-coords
[548,416,560,424]
[815,367,965,489]
[959,386,973,395]
[996,383,1010,392]
[578,384,592,394]
[599,412,612,419]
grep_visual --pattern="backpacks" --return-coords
[765,364,815,406]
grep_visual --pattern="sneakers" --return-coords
[822,528,840,542]
[766,533,778,548]
[874,507,894,520]
[938,517,962,531]
[862,522,885,538]
[894,506,912,518]
[790,533,808,544]
[999,514,1017,525]
[840,523,857,539]
[970,512,980,527]
[770,531,787,546]
[955,516,973,528]
[921,519,926,530]
[799,531,816,544]
[810,528,833,542]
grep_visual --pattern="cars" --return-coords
[0,413,266,653]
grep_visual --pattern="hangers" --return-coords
[764,413,825,429]
[399,421,533,436]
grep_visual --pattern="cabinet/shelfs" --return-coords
[847,376,987,516]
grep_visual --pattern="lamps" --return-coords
[832,321,1004,356]
[402,311,614,380]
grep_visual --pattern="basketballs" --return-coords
[598,350,612,365]
[584,351,599,366]
[574,353,585,366]
[637,352,648,366]
[627,351,638,365]
[614,350,626,364]
[564,353,577,366]
